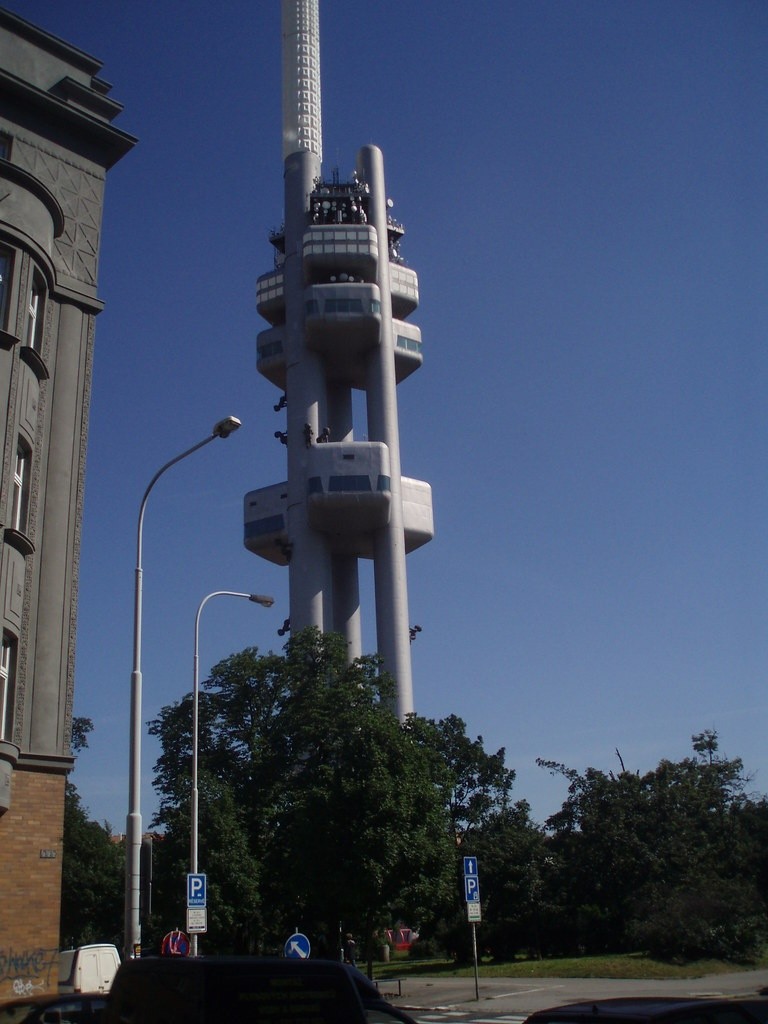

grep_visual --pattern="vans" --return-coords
[114,955,421,1024]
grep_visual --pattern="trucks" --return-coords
[58,943,121,998]
[384,928,416,951]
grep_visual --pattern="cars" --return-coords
[523,993,768,1024]
[0,994,112,1023]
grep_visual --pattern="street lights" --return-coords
[120,416,240,962]
[188,586,274,959]
